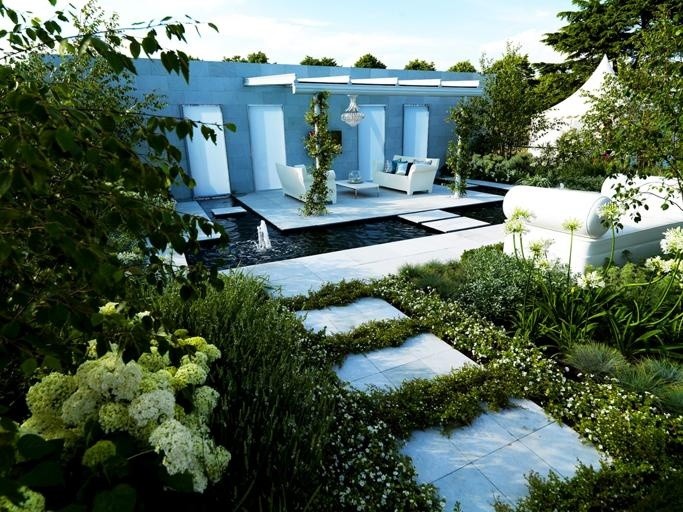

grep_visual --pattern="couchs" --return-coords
[370,154,440,196]
[276,164,336,204]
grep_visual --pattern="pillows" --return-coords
[384,158,432,175]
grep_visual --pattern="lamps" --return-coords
[341,94,366,127]
